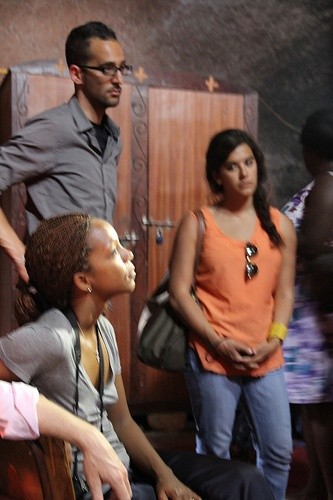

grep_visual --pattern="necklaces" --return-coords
[76,321,103,365]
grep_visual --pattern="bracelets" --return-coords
[213,338,226,349]
[268,322,286,341]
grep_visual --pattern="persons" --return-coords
[168,129,298,500]
[0,380,135,500]
[0,213,274,500]
[277,108,333,500]
[0,21,126,325]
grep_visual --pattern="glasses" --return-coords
[78,65,132,76]
[243,241,258,279]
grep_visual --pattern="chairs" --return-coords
[0,395,77,500]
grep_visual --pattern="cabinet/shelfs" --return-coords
[0,62,258,417]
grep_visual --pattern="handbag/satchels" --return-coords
[135,209,206,372]
